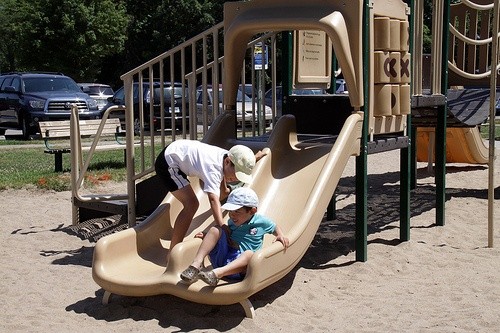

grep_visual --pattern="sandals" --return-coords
[198,270,219,287]
[180,265,203,283]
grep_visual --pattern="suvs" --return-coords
[0,71,99,141]
[100,82,190,135]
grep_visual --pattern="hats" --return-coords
[220,187,259,210]
[227,145,256,184]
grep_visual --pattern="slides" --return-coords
[92,111,362,319]
[416,125,490,165]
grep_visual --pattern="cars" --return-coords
[254,85,326,120]
[239,84,258,99]
[195,88,273,128]
[75,83,116,110]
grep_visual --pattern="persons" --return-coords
[155,139,256,252]
[181,188,289,287]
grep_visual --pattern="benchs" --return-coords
[38,118,140,173]
